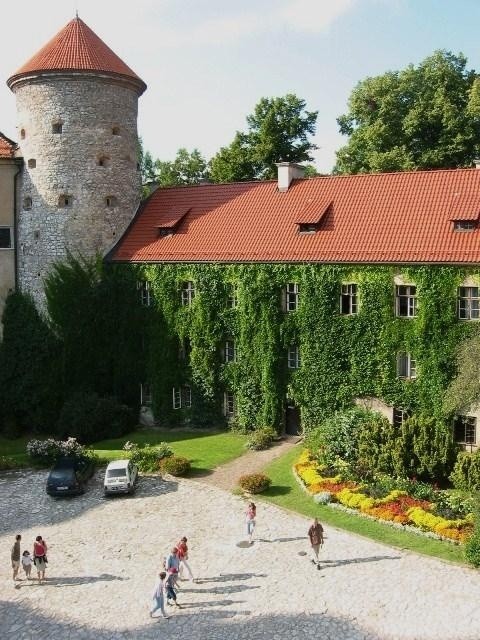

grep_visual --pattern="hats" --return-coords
[168,567,178,575]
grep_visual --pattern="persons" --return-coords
[21,551,34,580]
[166,548,180,587]
[175,536,196,583]
[148,571,170,619]
[10,535,24,582]
[244,502,257,544]
[32,536,48,582]
[164,567,182,610]
[307,517,326,570]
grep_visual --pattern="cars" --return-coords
[104,459,139,498]
[47,458,95,499]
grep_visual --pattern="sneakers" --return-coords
[312,558,320,570]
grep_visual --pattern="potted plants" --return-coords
[239,474,272,494]
[162,456,190,476]
